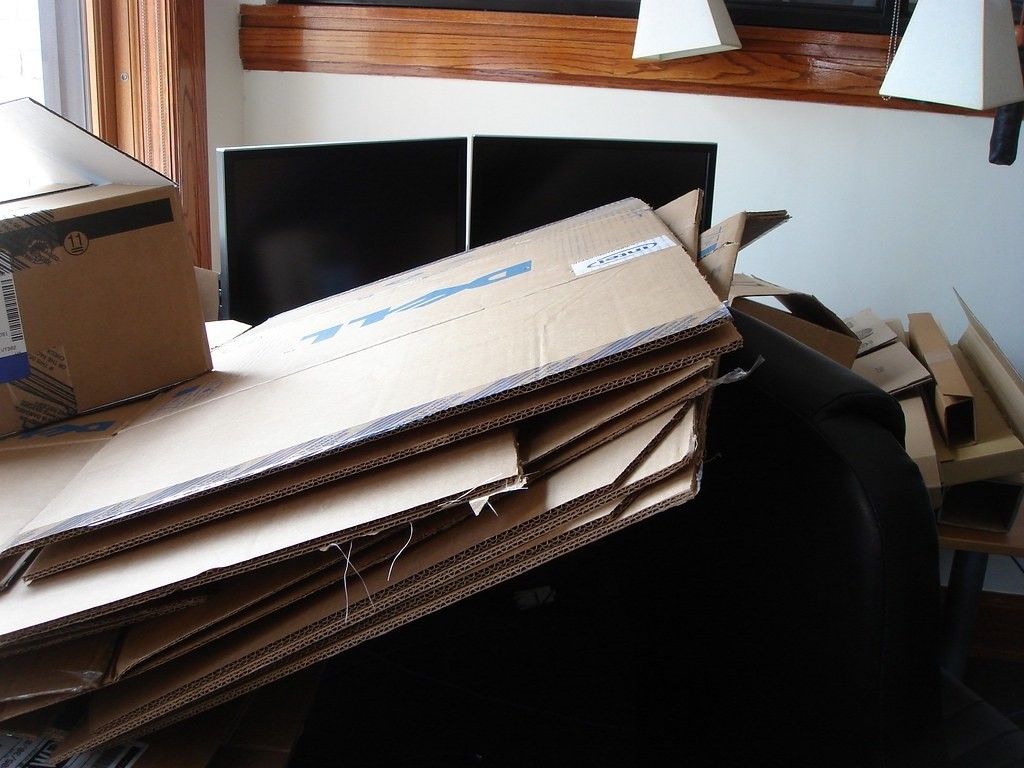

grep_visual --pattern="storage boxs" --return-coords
[730,273,862,370]
[0,96,215,442]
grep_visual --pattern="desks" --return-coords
[912,455,1024,680]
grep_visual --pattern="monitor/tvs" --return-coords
[471,134,716,251]
[218,137,469,330]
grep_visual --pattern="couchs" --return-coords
[291,312,1024,768]
